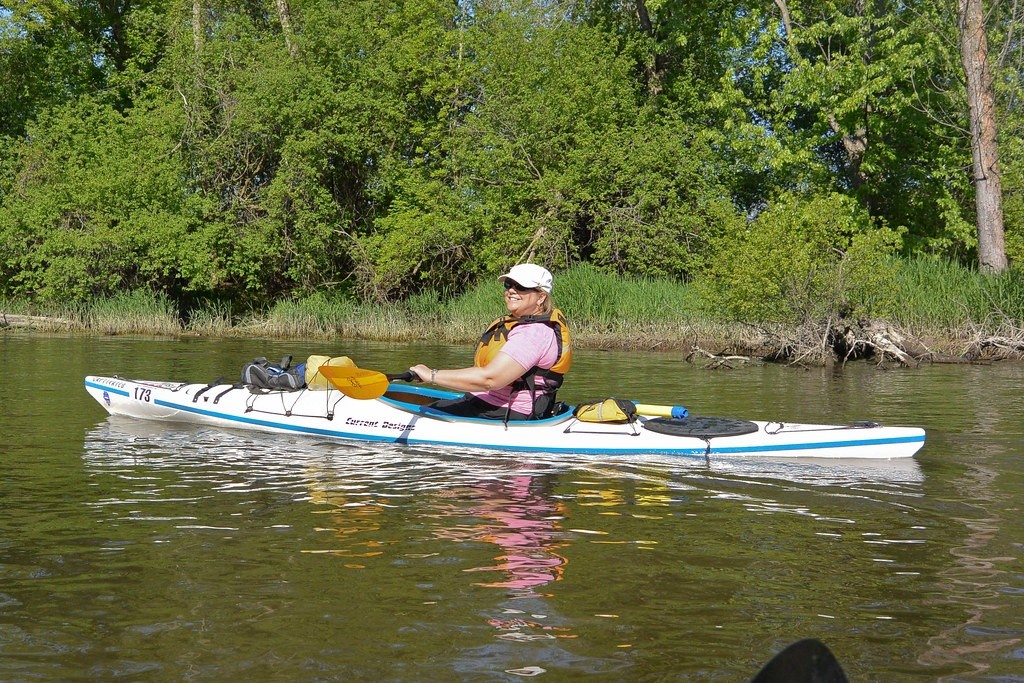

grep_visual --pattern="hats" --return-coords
[497,264,552,293]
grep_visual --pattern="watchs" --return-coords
[431,368,439,386]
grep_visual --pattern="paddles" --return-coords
[319,366,471,400]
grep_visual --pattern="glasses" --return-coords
[504,281,540,292]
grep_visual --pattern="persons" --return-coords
[409,262,573,421]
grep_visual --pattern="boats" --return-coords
[85,373,925,460]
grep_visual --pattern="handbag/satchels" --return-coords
[242,356,306,391]
